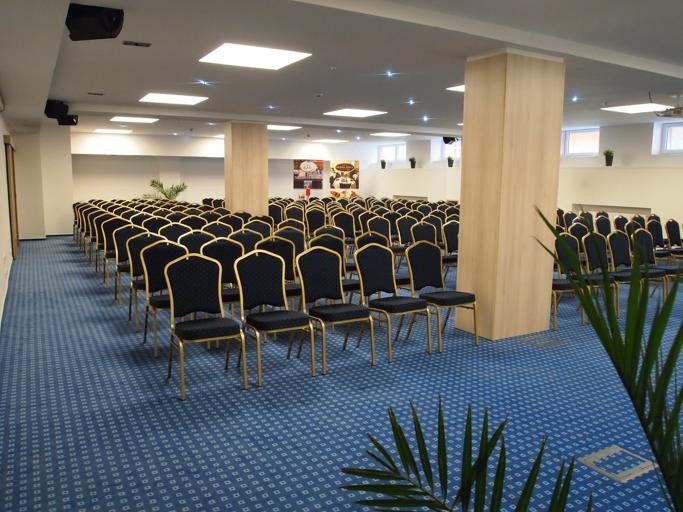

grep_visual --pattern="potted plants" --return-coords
[380,160,385,169]
[447,157,453,167]
[409,157,416,169]
[603,150,614,167]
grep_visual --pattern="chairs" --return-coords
[73,195,480,402]
[552,208,683,329]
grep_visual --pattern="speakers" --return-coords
[65,3,124,41]
[59,115,78,126]
[443,137,455,144]
